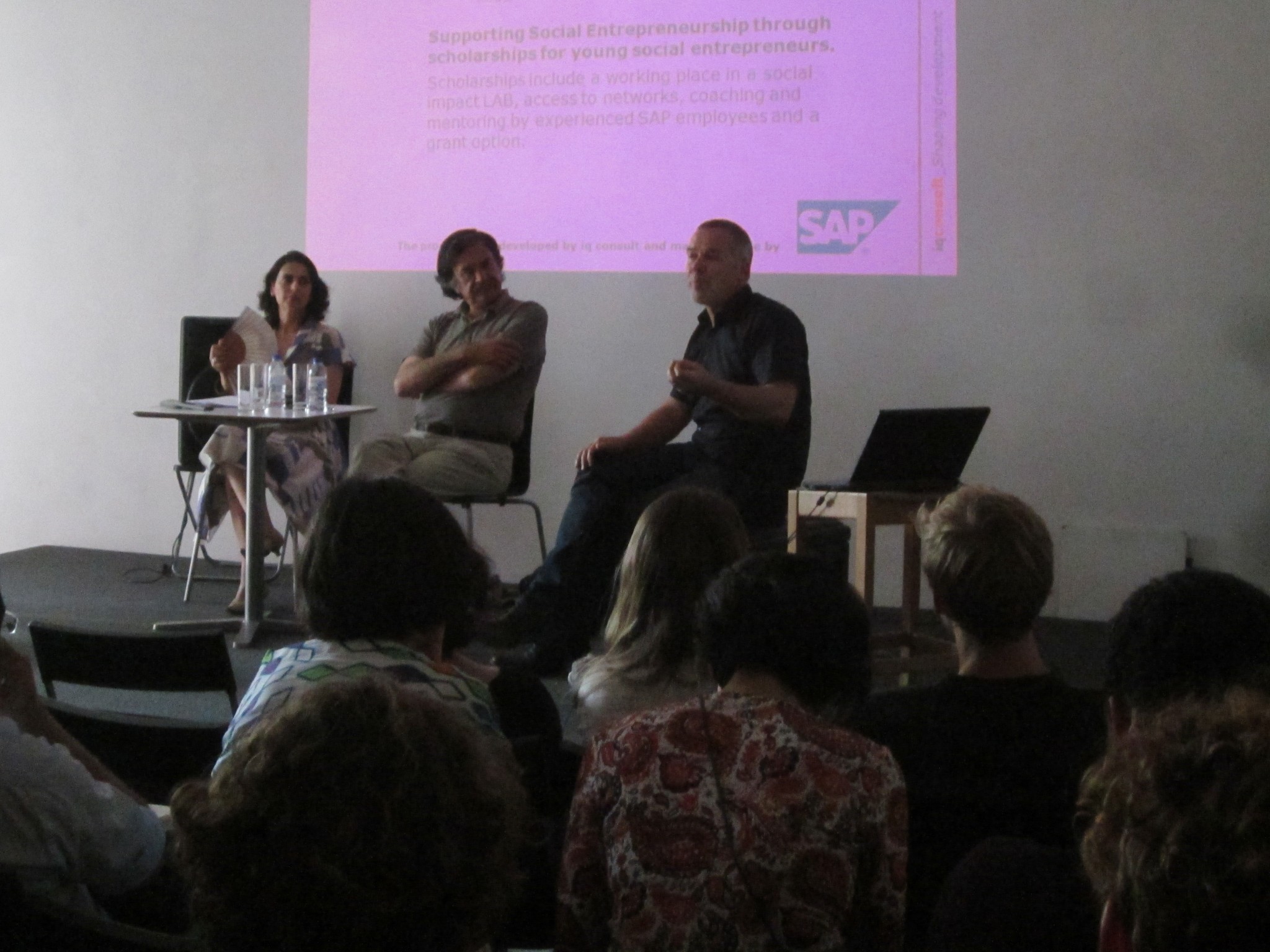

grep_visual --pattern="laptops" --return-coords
[801,407,991,492]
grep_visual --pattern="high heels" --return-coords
[241,528,283,559]
[226,581,266,613]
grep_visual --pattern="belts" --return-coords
[416,420,513,447]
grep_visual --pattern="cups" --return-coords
[238,362,251,412]
[248,363,268,411]
[291,362,308,410]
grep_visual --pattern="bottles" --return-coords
[268,355,286,411]
[310,355,325,411]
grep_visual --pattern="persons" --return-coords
[0,595,192,951]
[173,678,527,952]
[1101,570,1269,752]
[435,536,569,890]
[565,486,767,762]
[203,475,529,828]
[1074,688,1267,951]
[346,229,548,497]
[552,550,911,951]
[197,251,359,609]
[473,220,814,672]
[845,487,1110,951]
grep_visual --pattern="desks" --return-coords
[144,399,380,647]
[789,487,967,651]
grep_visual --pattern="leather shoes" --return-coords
[492,648,561,675]
[474,612,526,647]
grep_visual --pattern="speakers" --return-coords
[177,316,240,468]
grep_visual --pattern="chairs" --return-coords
[181,363,356,615]
[27,614,239,805]
[449,387,550,568]
